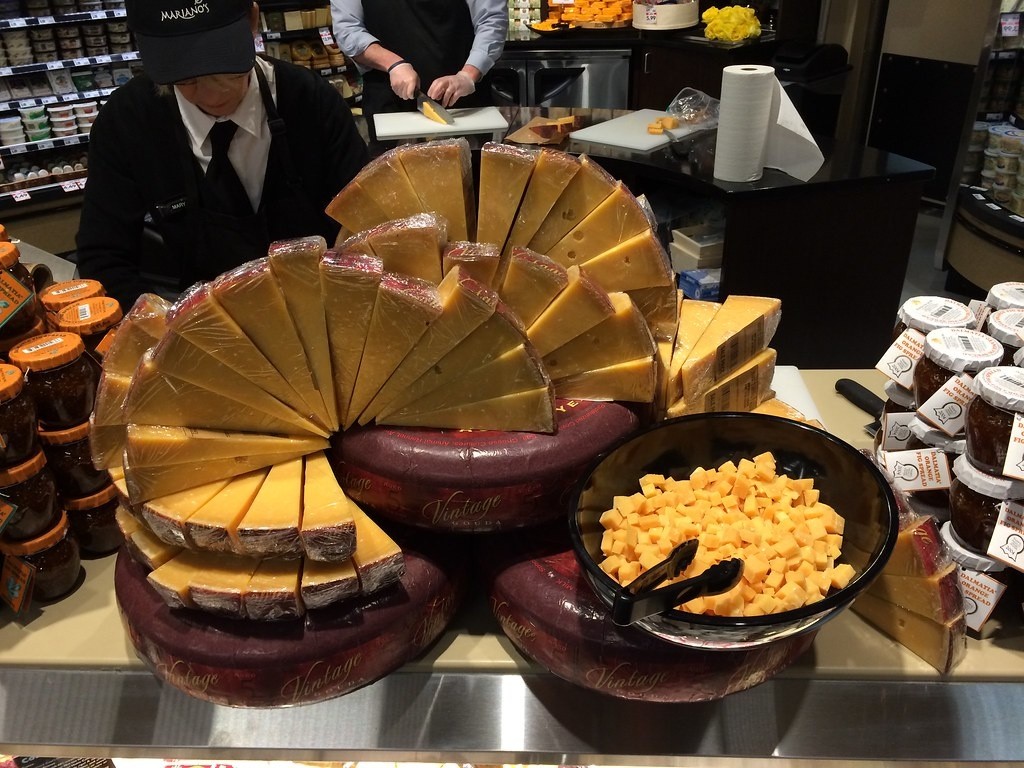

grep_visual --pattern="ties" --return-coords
[205,120,254,213]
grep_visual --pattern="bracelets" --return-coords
[388,60,414,74]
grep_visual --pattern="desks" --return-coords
[0,370,1024,762]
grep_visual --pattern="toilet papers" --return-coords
[711,63,827,184]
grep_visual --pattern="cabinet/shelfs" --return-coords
[0,0,365,223]
[934,0,1024,300]
[639,29,776,112]
[354,107,937,370]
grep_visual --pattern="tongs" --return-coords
[609,537,745,629]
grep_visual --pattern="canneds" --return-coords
[0,222,123,605]
[879,283,1024,638]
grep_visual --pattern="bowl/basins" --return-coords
[565,409,901,654]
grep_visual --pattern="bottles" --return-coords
[0,222,126,603]
[873,281,1024,612]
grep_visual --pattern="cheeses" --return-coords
[84,141,966,711]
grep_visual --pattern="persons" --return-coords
[330,0,509,157]
[75,0,372,315]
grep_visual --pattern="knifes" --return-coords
[662,127,688,157]
[835,373,888,437]
[413,81,455,125]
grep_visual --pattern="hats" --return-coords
[125,0,256,85]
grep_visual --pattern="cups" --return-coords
[0,18,141,68]
[0,0,124,20]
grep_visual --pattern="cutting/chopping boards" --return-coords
[372,106,509,138]
[569,108,704,151]
